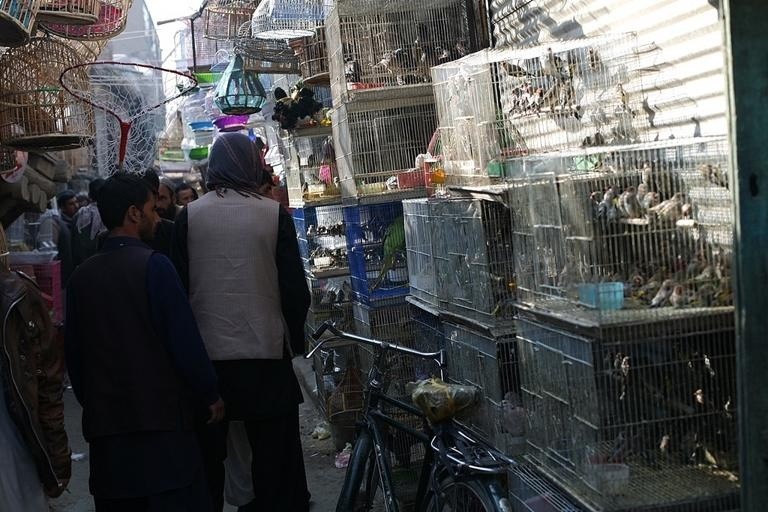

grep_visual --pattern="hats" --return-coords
[158,176,176,191]
[56,190,75,202]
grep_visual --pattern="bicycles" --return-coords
[306,320,518,512]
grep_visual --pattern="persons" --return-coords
[58,171,232,512]
[0,223,72,511]
[168,132,316,511]
[24,175,202,315]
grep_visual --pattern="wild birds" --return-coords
[600,350,734,463]
[341,20,470,87]
[481,46,603,117]
[580,79,654,147]
[585,156,698,219]
[621,235,736,312]
